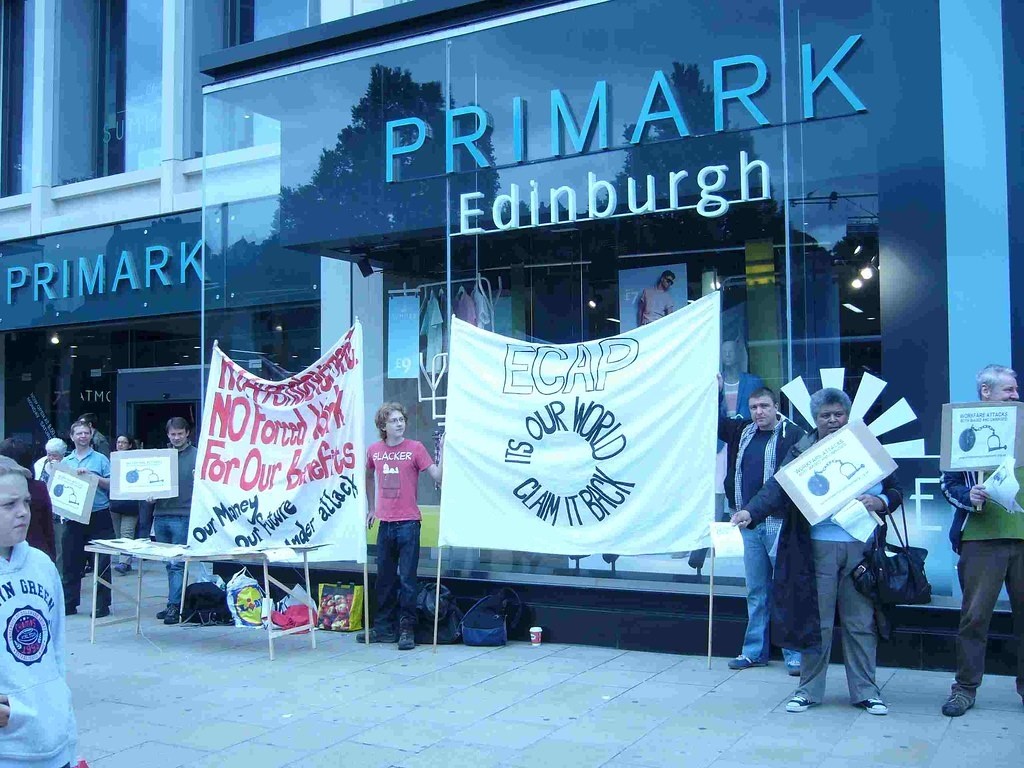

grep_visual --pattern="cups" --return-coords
[528,627,543,646]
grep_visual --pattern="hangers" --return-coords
[473,276,478,293]
[439,281,445,296]
[431,282,434,296]
[457,278,465,296]
[424,283,427,298]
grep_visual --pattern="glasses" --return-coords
[663,276,675,286]
[385,416,406,424]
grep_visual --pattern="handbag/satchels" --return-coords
[181,567,363,634]
[458,588,525,646]
[849,489,931,605]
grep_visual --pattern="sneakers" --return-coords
[164,603,180,624]
[355,629,398,643]
[65,605,77,615]
[941,692,975,716]
[727,655,766,668]
[781,648,802,675]
[852,699,888,715]
[156,611,167,619]
[785,696,818,712]
[397,627,415,649]
[90,606,110,617]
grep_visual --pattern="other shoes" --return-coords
[114,563,132,575]
[79,570,85,578]
[84,565,92,572]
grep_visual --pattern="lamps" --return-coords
[843,236,863,255]
[851,257,878,289]
[568,555,590,568]
[161,391,170,400]
[602,554,620,571]
[51,332,60,344]
[688,547,710,576]
[588,284,603,309]
[710,276,721,290]
[357,258,374,278]
[11,332,17,341]
[275,321,284,332]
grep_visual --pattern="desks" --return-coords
[85,536,335,662]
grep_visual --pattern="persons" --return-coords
[717,371,807,676]
[0,413,155,573]
[730,387,889,715]
[146,417,198,625]
[940,365,1024,716]
[0,455,79,768]
[60,421,116,618]
[714,340,765,523]
[356,401,446,649]
[637,270,673,327]
